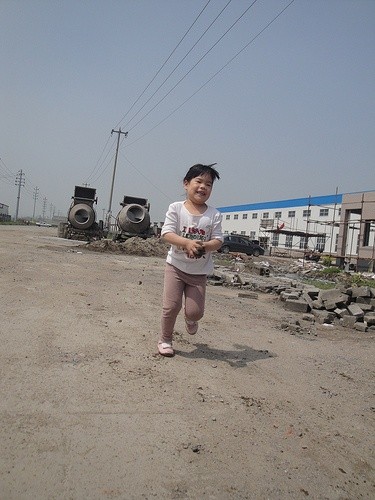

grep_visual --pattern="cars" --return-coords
[218,234,265,257]
[35,222,52,228]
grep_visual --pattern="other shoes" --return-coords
[157,338,173,357]
[183,312,198,334]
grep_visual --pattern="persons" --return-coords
[157,162,224,357]
